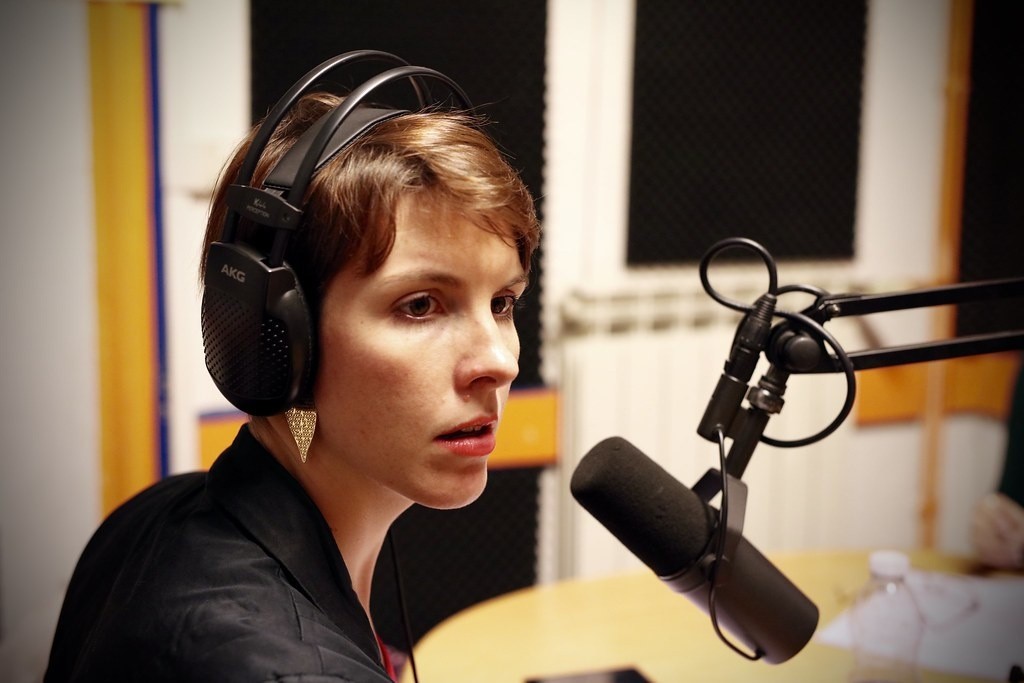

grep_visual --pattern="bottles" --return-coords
[846,550,923,682]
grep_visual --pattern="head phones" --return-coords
[203,48,482,419]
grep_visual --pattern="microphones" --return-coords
[565,439,820,668]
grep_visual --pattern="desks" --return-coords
[395,546,982,683]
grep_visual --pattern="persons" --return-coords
[967,360,1024,566]
[42,96,542,683]
